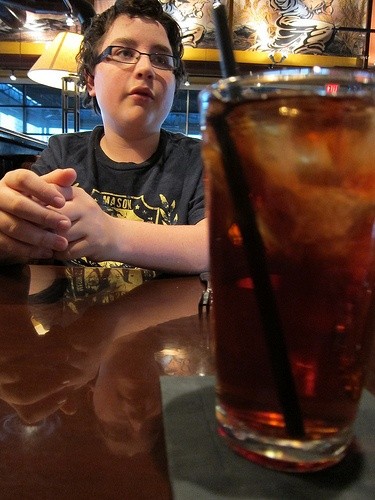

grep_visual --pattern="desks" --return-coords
[0,262,375,500]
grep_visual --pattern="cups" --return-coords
[197,73,375,477]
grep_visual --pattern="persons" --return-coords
[0,0,210,303]
[0,262,211,469]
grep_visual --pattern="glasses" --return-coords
[93,45,181,70]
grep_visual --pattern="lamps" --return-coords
[26,31,91,134]
